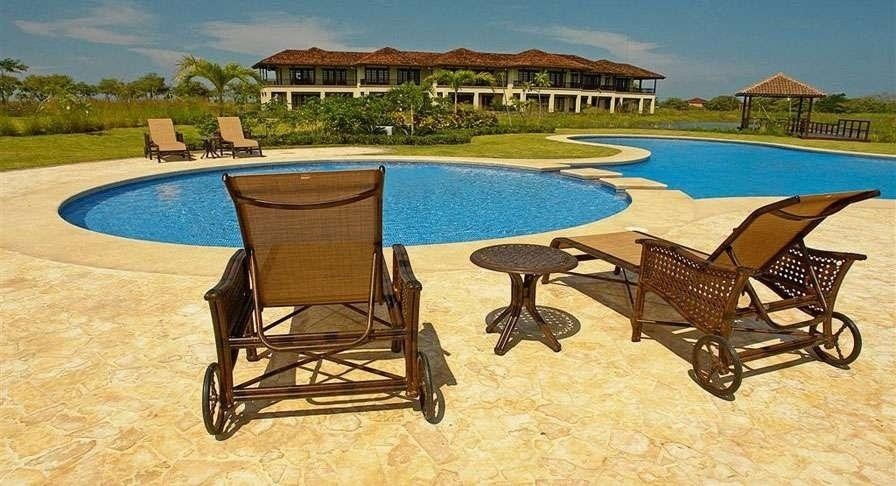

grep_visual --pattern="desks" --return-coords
[471,243,578,354]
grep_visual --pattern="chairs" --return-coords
[202,166,430,440]
[142,116,263,164]
[542,190,879,398]
